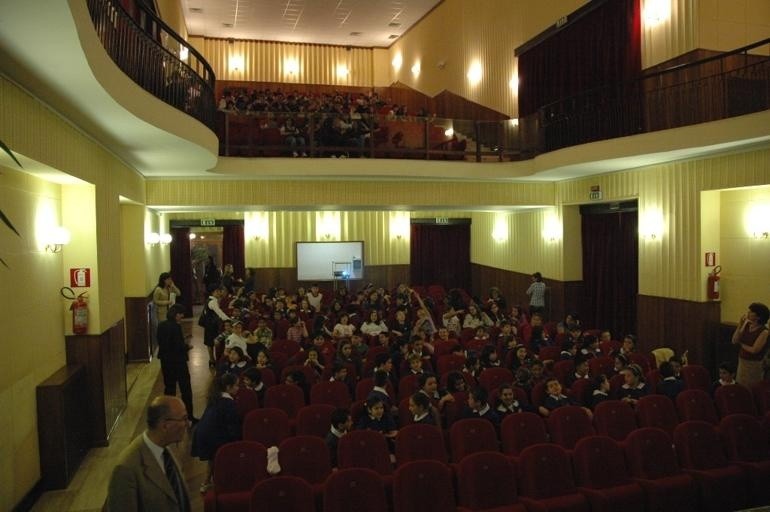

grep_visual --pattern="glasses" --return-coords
[166,415,190,423]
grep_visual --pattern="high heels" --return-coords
[209,360,215,367]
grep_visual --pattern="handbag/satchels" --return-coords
[199,313,213,329]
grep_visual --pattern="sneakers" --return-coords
[200,482,213,493]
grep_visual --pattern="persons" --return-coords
[196,254,739,492]
[153,272,182,321]
[166,71,428,158]
[100,395,192,511]
[157,303,199,422]
[731,303,770,388]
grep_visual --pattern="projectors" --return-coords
[335,270,349,277]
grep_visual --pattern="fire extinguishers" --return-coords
[706,266,724,301]
[60,287,89,337]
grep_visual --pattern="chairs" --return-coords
[210,91,455,160]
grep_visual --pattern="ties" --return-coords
[163,450,181,512]
